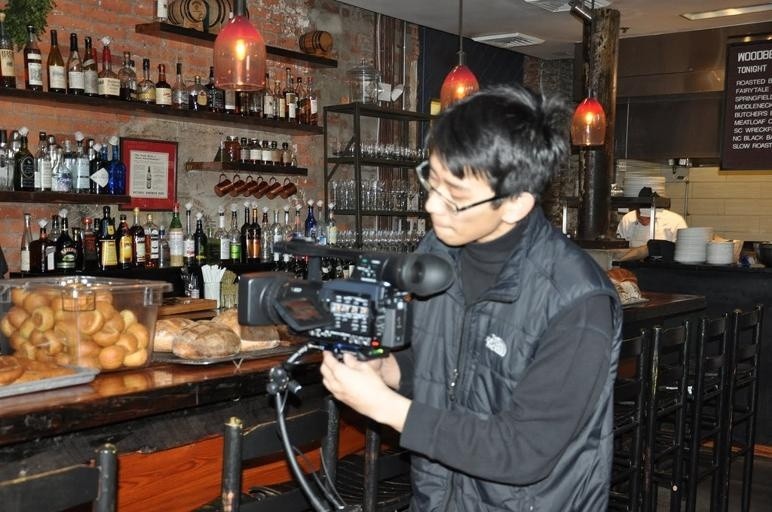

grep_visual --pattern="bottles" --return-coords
[1,127,124,196]
[306,76,319,127]
[275,80,285,120]
[208,66,224,112]
[189,76,208,110]
[264,72,274,119]
[286,67,299,127]
[249,72,263,118]
[49,28,66,97]
[155,64,172,105]
[741,242,760,265]
[322,256,354,279]
[346,58,384,109]
[138,58,155,103]
[226,135,298,166]
[610,183,624,196]
[24,24,42,95]
[20,201,337,272]
[236,90,249,117]
[119,50,138,101]
[82,36,98,99]
[99,35,120,100]
[172,62,189,109]
[296,76,307,124]
[67,33,85,98]
[0,10,16,91]
[225,68,235,114]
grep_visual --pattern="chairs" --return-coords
[635,312,731,512]
[190,395,340,512]
[381,438,412,463]
[608,327,650,512]
[314,385,413,512]
[674,303,768,512]
[614,319,692,512]
[1,441,120,511]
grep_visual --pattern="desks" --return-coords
[0,291,707,512]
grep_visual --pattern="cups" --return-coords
[183,276,239,312]
[215,174,297,199]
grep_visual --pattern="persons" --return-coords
[616,187,688,248]
[320,85,623,512]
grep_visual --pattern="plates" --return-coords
[673,226,736,266]
[623,170,665,199]
[750,264,765,268]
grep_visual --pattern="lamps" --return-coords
[438,0,480,112]
[212,0,268,91]
[569,0,607,149]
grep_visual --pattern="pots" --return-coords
[568,229,629,249]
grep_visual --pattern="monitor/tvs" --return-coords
[624,91,724,164]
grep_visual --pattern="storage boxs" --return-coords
[1,273,174,374]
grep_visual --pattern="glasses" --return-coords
[416,160,509,211]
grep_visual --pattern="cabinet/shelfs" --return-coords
[322,101,434,252]
[0,19,341,211]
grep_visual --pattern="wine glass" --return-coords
[334,140,430,256]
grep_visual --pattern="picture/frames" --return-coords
[117,135,179,213]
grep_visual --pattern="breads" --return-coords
[0,280,298,387]
